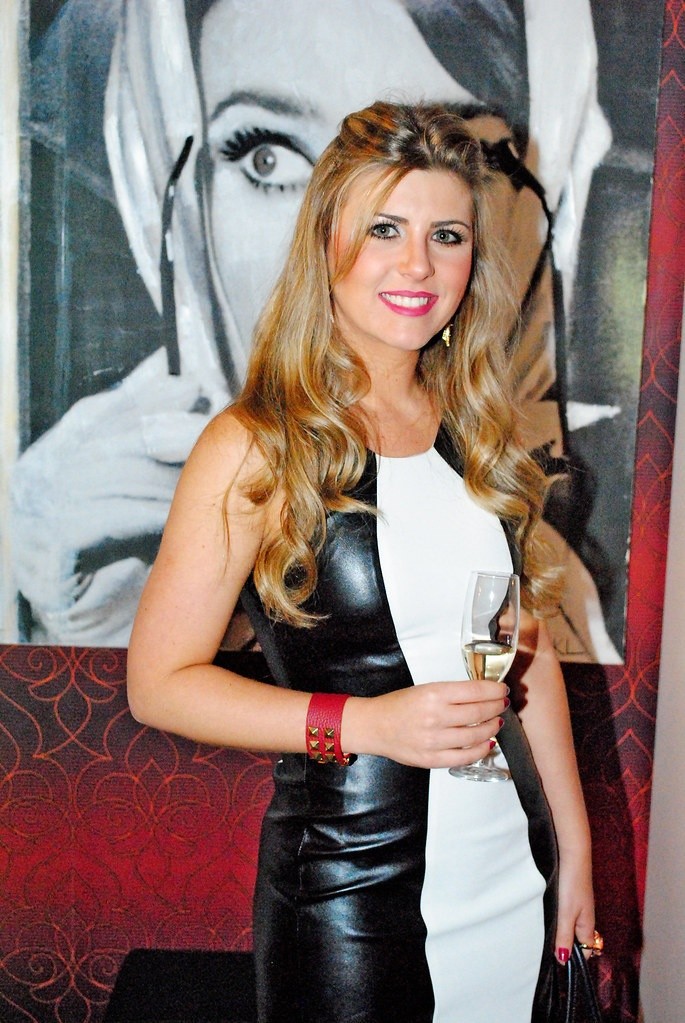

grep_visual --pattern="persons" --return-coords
[11,0,616,648]
[126,101,601,1023]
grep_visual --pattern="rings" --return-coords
[579,931,603,957]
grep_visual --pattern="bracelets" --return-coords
[306,693,351,766]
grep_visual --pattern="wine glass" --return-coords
[448,569,520,780]
